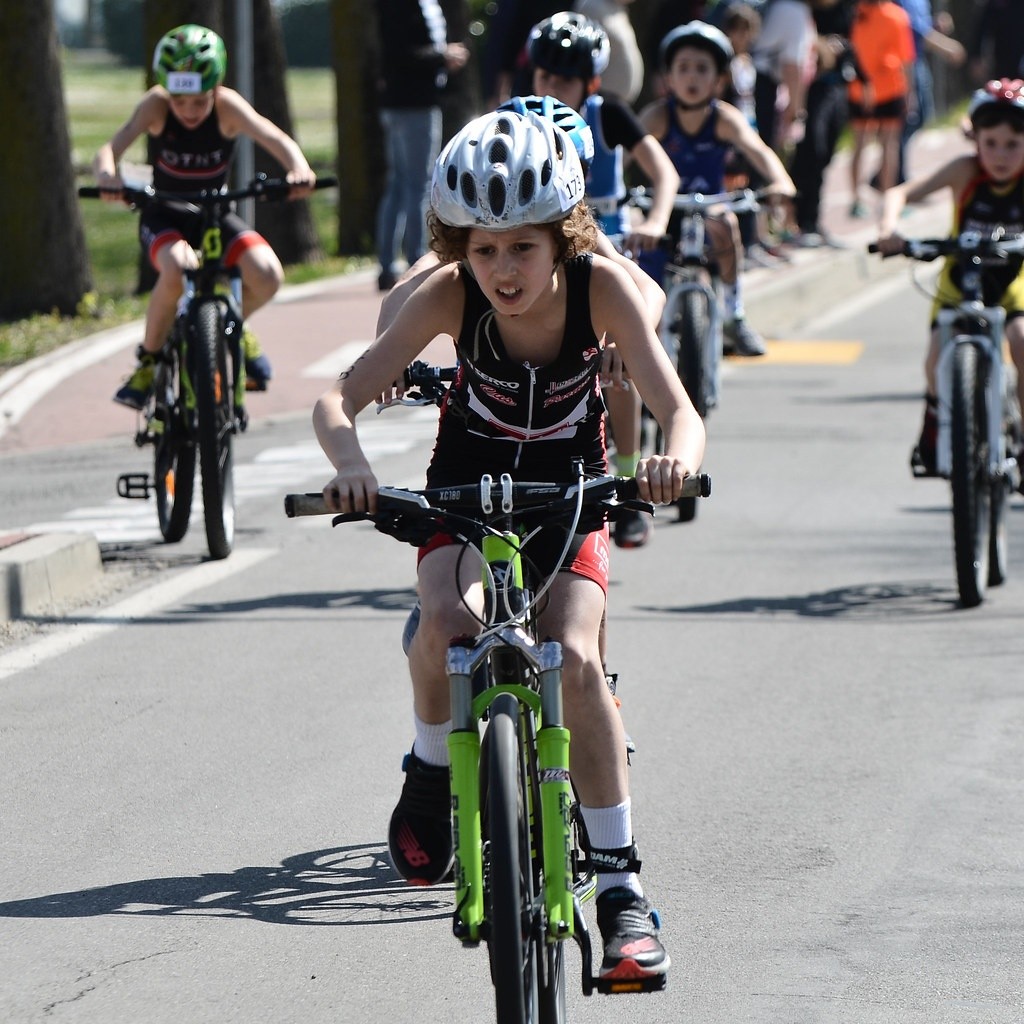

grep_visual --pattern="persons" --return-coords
[877,79,1024,466]
[373,94,667,656]
[623,23,797,354]
[94,24,316,407]
[373,1,645,290]
[311,110,704,980]
[526,12,681,549]
[710,1,960,267]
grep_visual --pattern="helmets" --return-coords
[966,78,1024,132]
[497,95,595,168]
[430,110,585,231]
[151,23,227,99]
[660,19,734,76]
[527,11,611,81]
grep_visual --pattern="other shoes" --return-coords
[613,508,649,548]
[723,317,764,357]
[741,222,837,274]
[919,407,942,474]
[379,272,403,291]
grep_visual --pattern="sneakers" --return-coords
[596,886,670,983]
[114,358,158,410]
[388,738,456,888]
[241,322,272,381]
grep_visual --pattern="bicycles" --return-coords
[284,361,713,1023]
[870,226,1024,608]
[590,186,769,523]
[73,171,342,562]
